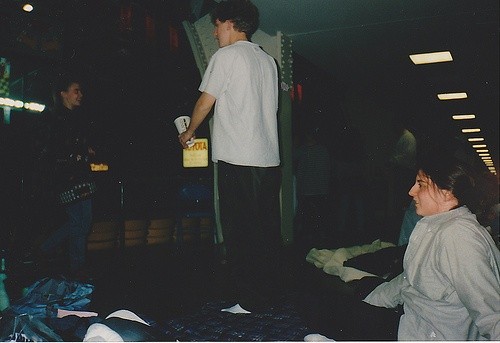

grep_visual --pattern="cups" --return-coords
[174,116,195,147]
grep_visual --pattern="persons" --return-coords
[178,1,280,315]
[398,169,499,248]
[50,76,100,283]
[353,149,500,341]
